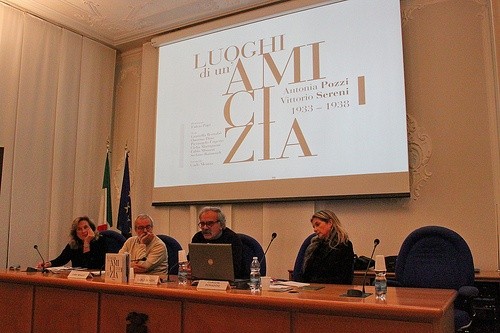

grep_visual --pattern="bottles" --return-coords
[374,271,386,302]
[178,262,188,284]
[250,257,260,291]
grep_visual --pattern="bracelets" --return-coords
[84,244,91,248]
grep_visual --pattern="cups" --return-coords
[178,250,188,262]
[374,255,386,271]
[261,277,271,290]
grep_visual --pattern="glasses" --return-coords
[135,225,152,231]
[198,220,220,229]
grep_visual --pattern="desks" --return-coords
[289,269,500,333]
[0,266,459,333]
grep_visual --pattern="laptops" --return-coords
[188,243,253,286]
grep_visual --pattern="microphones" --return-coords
[236,232,278,290]
[99,257,147,276]
[33,245,51,274]
[348,238,380,299]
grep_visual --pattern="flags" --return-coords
[97,152,113,232]
[116,153,132,238]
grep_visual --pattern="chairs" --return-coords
[397,225,477,333]
[156,234,182,275]
[293,234,318,283]
[237,233,267,276]
[100,231,129,255]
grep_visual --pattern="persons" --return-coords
[37,215,106,271]
[291,210,354,285]
[118,214,168,274]
[185,207,251,279]
